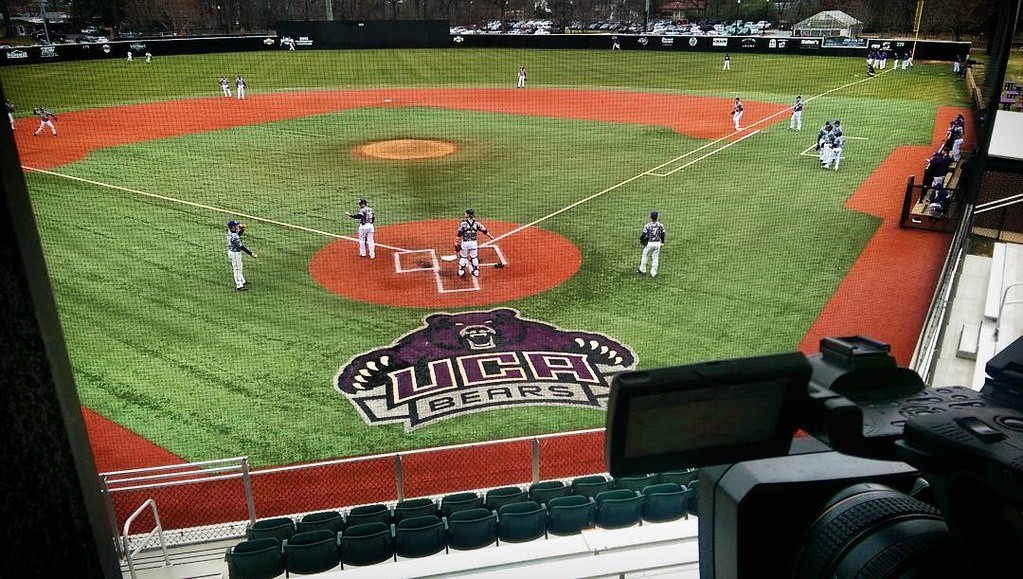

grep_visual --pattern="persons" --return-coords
[913,113,965,223]
[790,96,803,131]
[345,198,376,259]
[866,48,913,77]
[723,53,730,70]
[638,211,666,278]
[816,120,843,171]
[219,76,232,98]
[6,98,15,130]
[33,108,57,136]
[145,51,152,64]
[731,97,743,129]
[455,210,494,277]
[227,220,257,291]
[289,39,295,50]
[612,36,620,51]
[517,66,527,88]
[953,54,970,74]
[236,76,247,99]
[127,50,133,61]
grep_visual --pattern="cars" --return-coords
[0,26,180,50]
[449,17,774,36]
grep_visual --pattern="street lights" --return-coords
[570,1,574,34]
[735,0,742,36]
[762,0,770,34]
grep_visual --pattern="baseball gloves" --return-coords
[731,111,735,115]
[54,116,57,121]
[454,244,462,253]
[815,145,820,151]
[640,239,648,246]
[239,224,247,230]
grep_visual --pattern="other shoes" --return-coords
[244,281,251,284]
[239,286,248,291]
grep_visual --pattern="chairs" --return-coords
[482,485,527,513]
[392,516,448,563]
[282,530,342,579]
[443,509,498,555]
[636,483,690,527]
[435,491,483,518]
[543,494,596,541]
[657,468,699,485]
[391,497,439,525]
[245,510,346,544]
[224,537,284,579]
[566,474,611,495]
[609,471,659,491]
[337,522,396,571]
[685,480,701,520]
[525,480,572,500]
[592,490,642,532]
[344,503,393,531]
[493,501,547,548]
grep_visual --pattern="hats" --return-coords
[936,183,942,188]
[227,221,239,226]
[836,132,842,136]
[466,208,475,217]
[735,98,739,101]
[357,199,366,204]
[834,120,840,124]
[651,212,658,219]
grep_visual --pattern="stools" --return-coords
[1004,80,1018,90]
[1000,97,1016,111]
[1006,91,1019,99]
[1015,85,1023,94]
